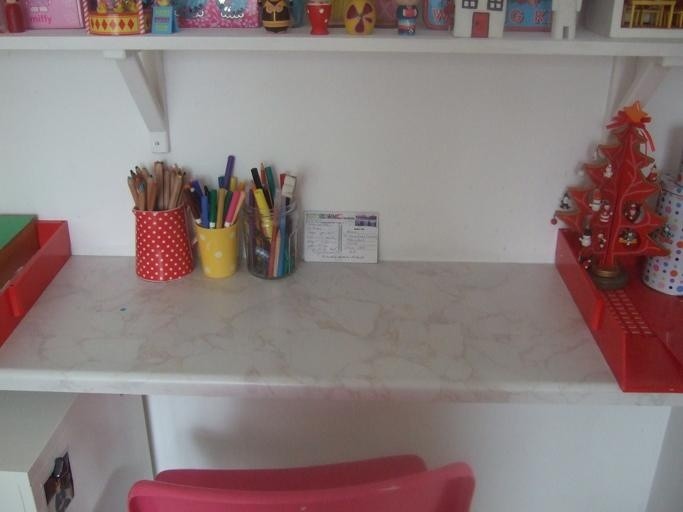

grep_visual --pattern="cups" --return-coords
[305,4,332,35]
[192,216,241,280]
[237,197,299,280]
[131,202,195,282]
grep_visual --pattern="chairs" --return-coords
[126,454,474,511]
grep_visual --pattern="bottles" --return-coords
[5,0,25,33]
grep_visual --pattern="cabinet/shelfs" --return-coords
[0,0,683,512]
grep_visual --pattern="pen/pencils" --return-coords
[216,176,224,188]
[265,167,275,206]
[261,162,271,212]
[127,161,185,211]
[247,181,255,265]
[222,191,240,228]
[200,195,208,229]
[224,156,234,190]
[203,186,210,198]
[189,186,200,209]
[207,189,217,229]
[277,214,285,278]
[190,179,203,197]
[215,189,227,228]
[280,173,286,189]
[273,227,281,276]
[224,191,232,219]
[231,191,246,225]
[253,188,272,243]
[229,178,237,191]
[237,183,245,190]
[249,168,262,190]
[182,186,201,227]
